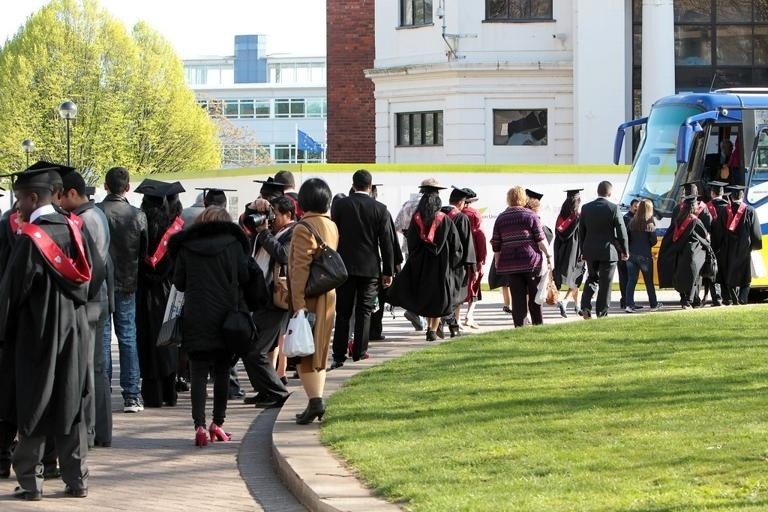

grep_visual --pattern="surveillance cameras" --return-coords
[552,32,565,40]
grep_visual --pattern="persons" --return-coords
[709,140,741,184]
[617,200,644,311]
[625,199,663,312]
[578,181,630,319]
[490,191,553,313]
[657,181,763,309]
[92,165,338,448]
[552,189,586,317]
[0,160,113,501]
[330,168,487,369]
[490,185,555,327]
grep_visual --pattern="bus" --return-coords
[616,86,768,289]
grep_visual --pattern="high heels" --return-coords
[176,375,189,392]
[349,341,354,357]
[209,423,232,442]
[556,301,567,318]
[296,404,310,418]
[503,306,513,316]
[195,426,208,448]
[296,405,325,424]
[574,306,580,315]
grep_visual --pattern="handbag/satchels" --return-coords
[221,309,259,355]
[292,221,348,298]
[155,315,183,347]
[272,263,289,310]
[702,251,719,279]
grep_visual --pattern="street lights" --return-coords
[59,102,78,168]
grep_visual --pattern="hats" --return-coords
[253,180,292,198]
[0,167,60,208]
[706,181,729,190]
[169,181,185,196]
[526,189,543,200]
[195,187,237,202]
[134,179,172,203]
[680,194,701,203]
[86,186,95,199]
[680,181,697,188]
[563,189,584,197]
[372,183,383,191]
[27,161,76,184]
[418,185,447,197]
[451,185,468,199]
[725,184,747,193]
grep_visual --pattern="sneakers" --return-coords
[650,302,663,312]
[123,398,145,413]
[624,306,636,313]
[405,312,479,342]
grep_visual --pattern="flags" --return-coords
[297,130,323,155]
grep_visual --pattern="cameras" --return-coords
[245,203,276,232]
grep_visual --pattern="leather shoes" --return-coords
[13,487,42,500]
[64,485,87,498]
[244,392,260,404]
[280,376,289,385]
[327,361,343,371]
[255,399,280,408]
[227,390,245,400]
[377,335,385,340]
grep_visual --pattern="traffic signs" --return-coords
[20,139,33,168]
